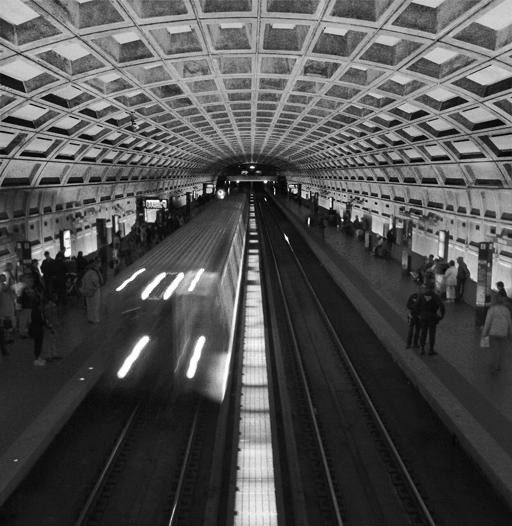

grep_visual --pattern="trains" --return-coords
[107,191,253,405]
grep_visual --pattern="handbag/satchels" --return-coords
[79,287,96,297]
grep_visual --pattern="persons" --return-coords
[293,192,512,372]
[1,192,214,366]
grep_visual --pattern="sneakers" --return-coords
[88,317,99,325]
[32,355,62,367]
[406,344,438,356]
[489,365,503,376]
